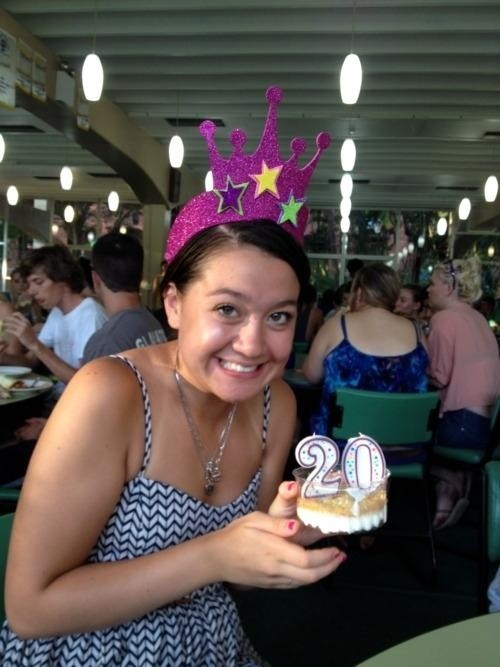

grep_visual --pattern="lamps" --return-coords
[340,111,357,172]
[339,174,354,199]
[338,1,364,105]
[81,1,104,102]
[58,133,74,192]
[436,217,449,238]
[168,95,185,169]
[417,210,427,248]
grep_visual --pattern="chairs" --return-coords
[433,393,499,533]
[484,458,500,562]
[322,387,441,570]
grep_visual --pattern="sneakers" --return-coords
[431,470,470,536]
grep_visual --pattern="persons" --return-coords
[389,282,422,317]
[0,87,375,667]
[409,257,500,532]
[0,244,108,484]
[149,260,166,296]
[302,264,430,554]
[334,259,367,304]
[303,286,334,343]
[1,267,28,306]
[0,232,168,440]
[338,285,353,306]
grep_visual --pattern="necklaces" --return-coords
[173,366,239,494]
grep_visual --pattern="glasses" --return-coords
[443,259,456,291]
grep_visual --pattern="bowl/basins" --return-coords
[291,464,391,536]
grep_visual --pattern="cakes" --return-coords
[295,470,388,538]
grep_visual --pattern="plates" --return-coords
[5,378,52,394]
[0,366,32,377]
